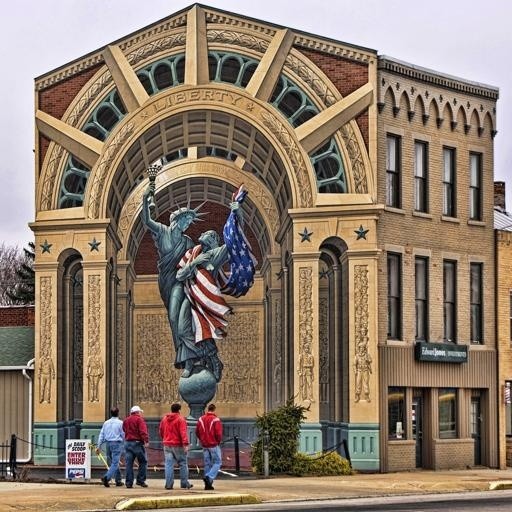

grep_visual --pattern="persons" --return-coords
[175,201,244,381]
[140,182,197,378]
[159,403,193,490]
[195,404,224,490]
[86,350,103,402]
[298,345,316,403]
[353,345,371,403]
[37,350,54,404]
[93,407,126,488]
[122,406,149,488]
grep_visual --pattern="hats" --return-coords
[130,406,143,414]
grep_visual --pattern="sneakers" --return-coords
[101,475,148,488]
[181,484,193,488]
[202,476,214,490]
